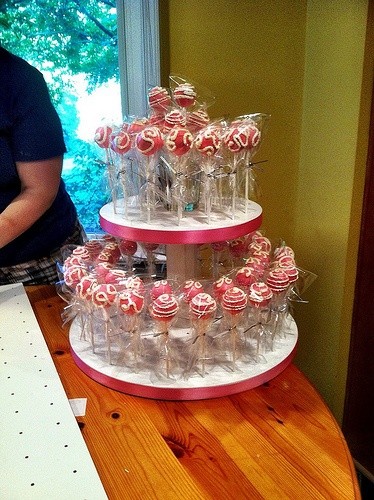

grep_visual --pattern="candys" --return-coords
[62,235,299,380]
[94,82,262,227]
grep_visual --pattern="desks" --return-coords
[0,285,362,500]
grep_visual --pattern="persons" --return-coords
[0,46,89,285]
